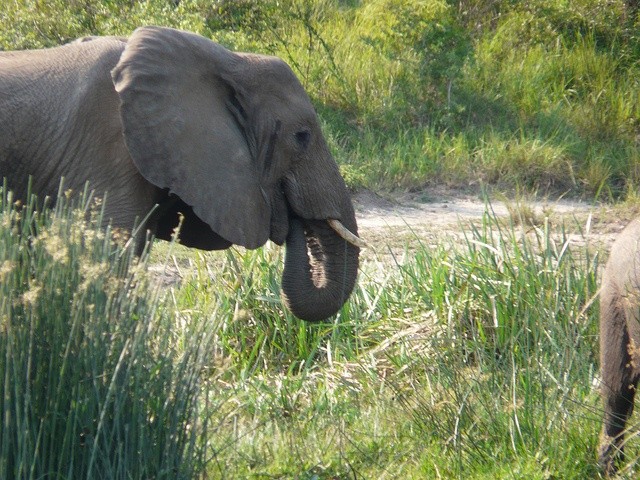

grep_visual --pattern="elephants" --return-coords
[595,213,640,478]
[0,26,369,321]
